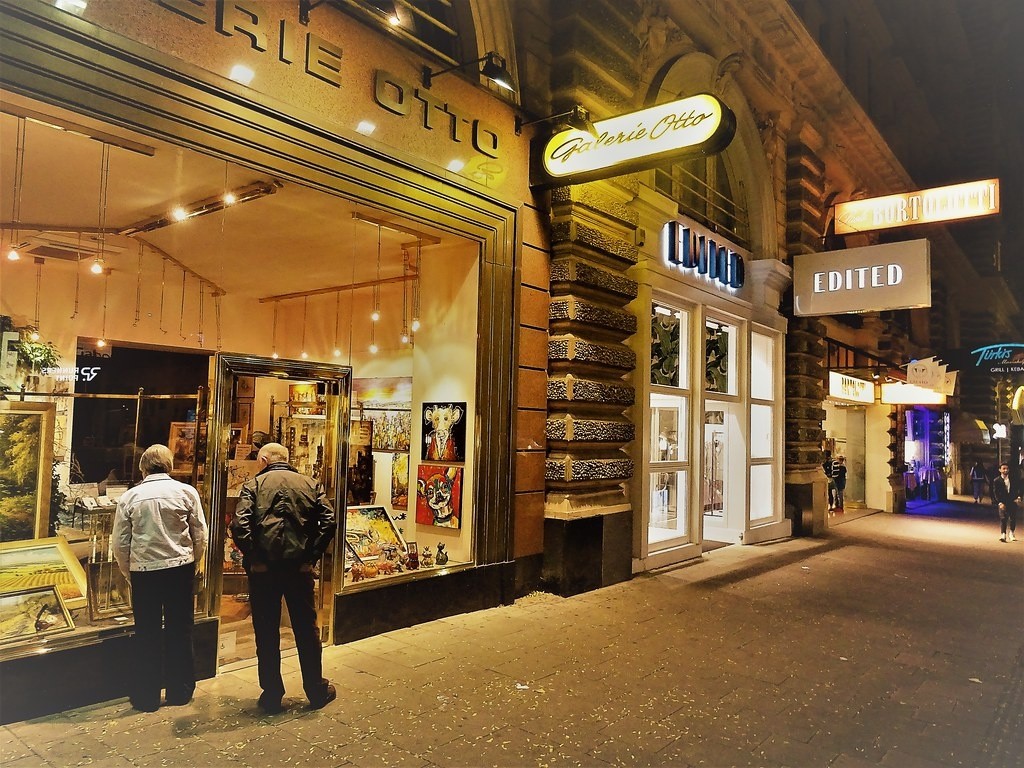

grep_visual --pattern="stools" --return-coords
[233,565,249,602]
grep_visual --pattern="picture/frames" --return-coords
[0,584,75,645]
[345,504,408,568]
[168,422,248,476]
[235,375,255,398]
[0,400,56,543]
[0,536,89,610]
[238,403,251,430]
[85,560,133,622]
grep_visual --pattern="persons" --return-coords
[231,443,337,714]
[98,442,146,496]
[970,458,1024,542]
[822,449,847,512]
[112,444,209,713]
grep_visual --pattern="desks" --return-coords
[72,497,117,531]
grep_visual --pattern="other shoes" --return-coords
[1008,533,1018,541]
[829,508,833,511]
[999,532,1006,541]
[256,683,287,709]
[310,684,336,710]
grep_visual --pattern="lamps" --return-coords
[423,51,518,94]
[298,0,400,26]
[515,105,600,140]
